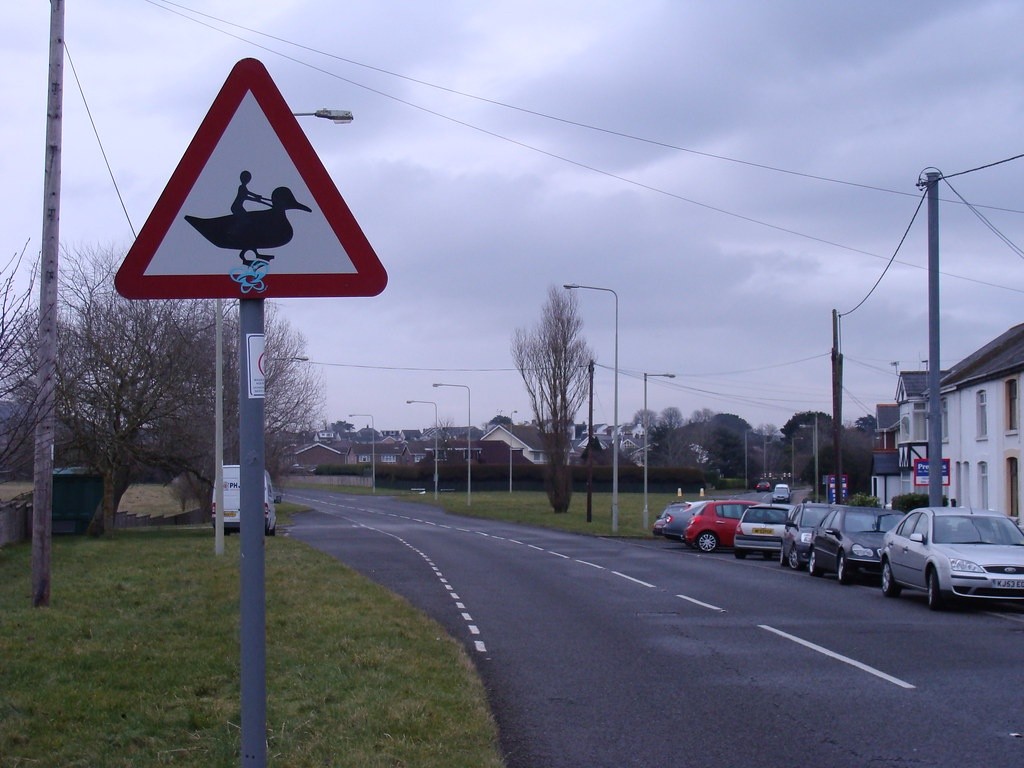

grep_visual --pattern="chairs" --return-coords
[762,513,771,523]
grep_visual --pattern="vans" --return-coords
[211,464,281,535]
[775,484,789,490]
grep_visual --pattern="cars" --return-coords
[734,504,797,561]
[652,502,708,542]
[878,506,1024,610]
[780,503,843,570]
[756,482,771,492]
[681,500,762,554]
[806,506,905,585]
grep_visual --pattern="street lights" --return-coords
[432,384,471,506]
[791,436,804,491]
[217,107,353,557]
[563,283,620,531]
[407,400,438,499]
[509,411,518,492]
[800,424,818,503]
[349,415,375,494]
[643,373,676,528]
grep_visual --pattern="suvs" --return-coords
[772,490,789,503]
